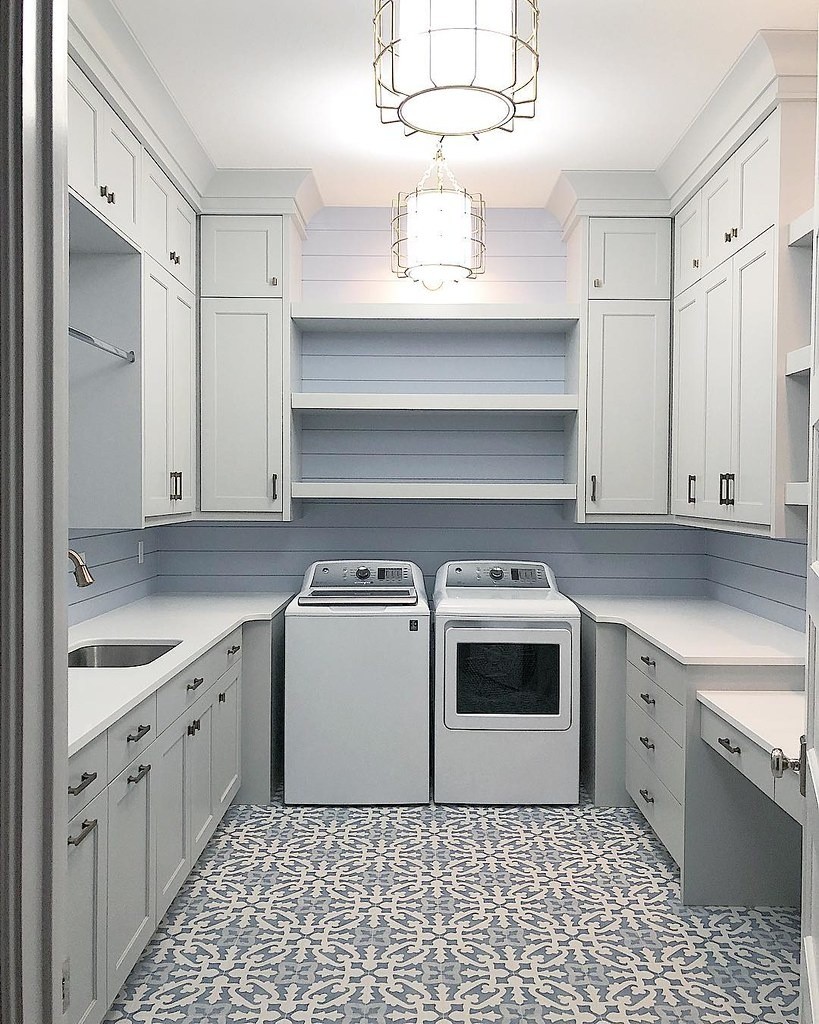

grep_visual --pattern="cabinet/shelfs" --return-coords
[572,211,671,527]
[184,628,246,862]
[67,730,108,1024]
[59,151,209,533]
[622,627,804,870]
[109,672,186,1012]
[778,208,816,544]
[57,51,133,262]
[198,210,298,524]
[676,157,739,531]
[291,297,577,501]
[734,106,783,539]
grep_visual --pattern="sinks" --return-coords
[67,638,184,667]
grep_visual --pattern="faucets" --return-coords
[67,548,95,588]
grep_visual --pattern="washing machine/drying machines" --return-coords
[284,559,429,807]
[433,559,581,807]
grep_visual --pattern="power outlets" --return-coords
[138,540,144,565]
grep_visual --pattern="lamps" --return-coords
[392,143,487,288]
[372,0,541,142]
